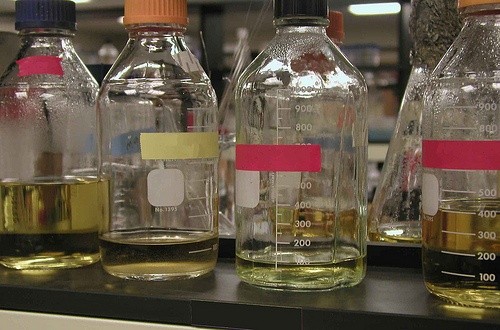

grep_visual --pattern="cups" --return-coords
[366,63,438,245]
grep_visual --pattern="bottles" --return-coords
[95,0,221,284]
[326,9,352,59]
[233,0,368,293]
[0,0,111,276]
[420,0,500,330]
